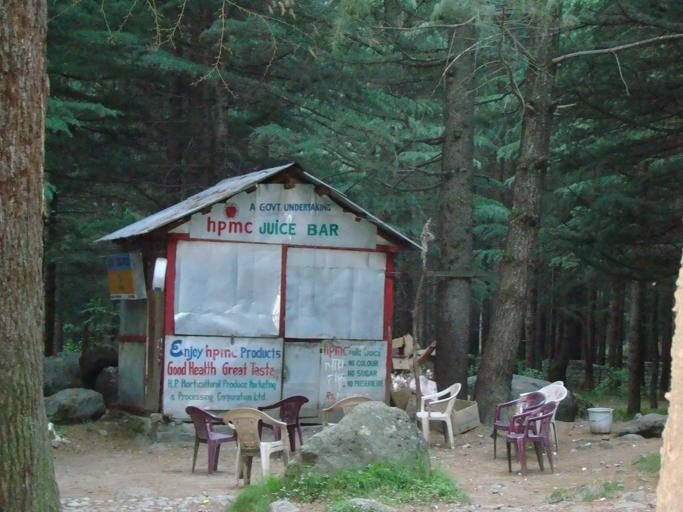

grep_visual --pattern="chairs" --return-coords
[414,376,456,447]
[489,379,570,473]
[184,392,374,487]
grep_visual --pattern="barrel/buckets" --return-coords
[587,408,614,433]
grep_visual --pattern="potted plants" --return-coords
[575,373,615,435]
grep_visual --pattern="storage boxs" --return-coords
[432,397,483,437]
[388,333,415,410]
